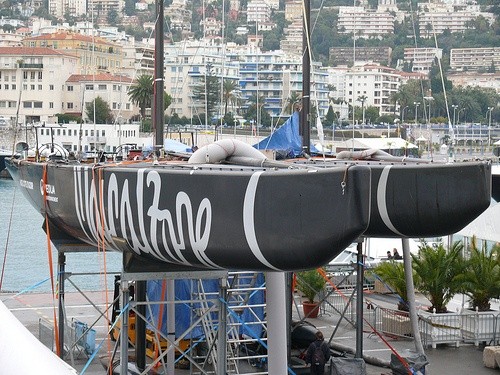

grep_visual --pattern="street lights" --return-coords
[458,108,466,126]
[413,101,421,124]
[487,107,494,146]
[402,105,409,123]
[260,102,268,125]
[423,96,435,159]
[190,99,200,127]
[451,105,458,128]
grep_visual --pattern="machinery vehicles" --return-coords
[107,275,210,371]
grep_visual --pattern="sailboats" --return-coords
[4,1,370,274]
[252,0,492,238]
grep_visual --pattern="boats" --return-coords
[324,235,449,289]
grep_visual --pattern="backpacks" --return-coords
[311,341,327,367]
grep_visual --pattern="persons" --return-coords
[304,331,330,375]
[393,248,402,260]
[387,250,393,260]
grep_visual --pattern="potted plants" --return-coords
[296,267,329,318]
[368,241,500,351]
